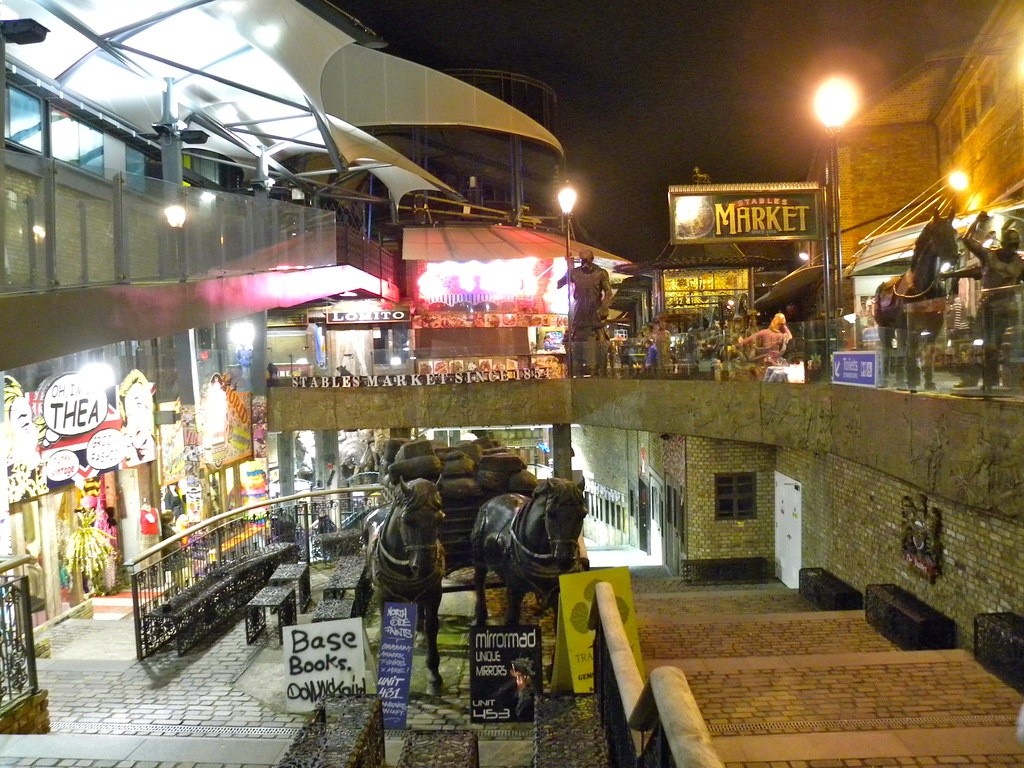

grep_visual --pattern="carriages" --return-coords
[361,439,589,695]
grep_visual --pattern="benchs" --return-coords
[865,583,957,651]
[143,575,237,657]
[209,542,300,608]
[268,564,311,614]
[799,568,863,610]
[311,598,357,623]
[311,529,363,567]
[322,556,370,617]
[245,586,298,646]
[972,611,1024,696]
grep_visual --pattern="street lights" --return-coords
[823,90,846,351]
[558,188,577,377]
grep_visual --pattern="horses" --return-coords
[470,475,591,686]
[874,209,960,394]
[362,471,448,699]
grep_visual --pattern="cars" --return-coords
[669,336,687,359]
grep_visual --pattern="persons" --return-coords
[618,342,625,368]
[609,342,616,369]
[738,314,793,379]
[562,250,612,377]
[939,211,1024,388]
[138,503,163,588]
[641,323,672,375]
[164,484,185,517]
[543,335,560,350]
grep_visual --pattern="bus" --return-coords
[427,426,553,448]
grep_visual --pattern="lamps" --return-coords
[151,122,177,136]
[269,187,291,197]
[180,130,210,144]
[0,18,51,45]
[233,187,254,195]
[250,179,267,191]
